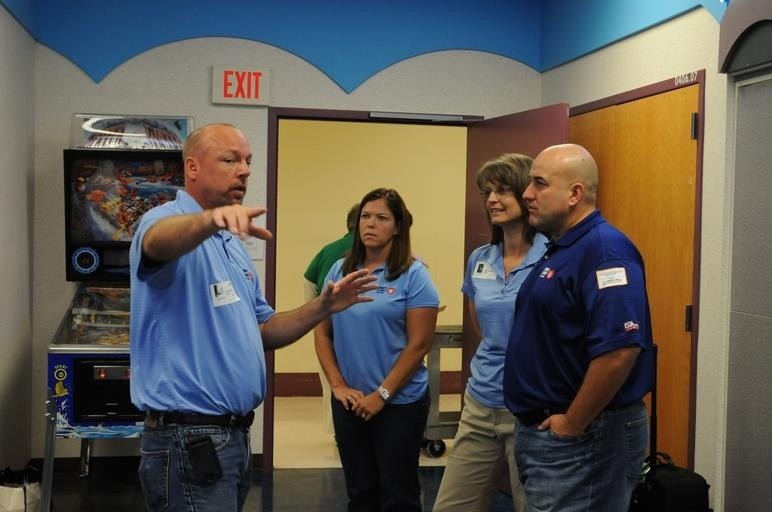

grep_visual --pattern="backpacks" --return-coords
[628,452,711,512]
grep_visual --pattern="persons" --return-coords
[130,123,378,512]
[435,143,527,512]
[502,144,655,511]
[303,189,440,512]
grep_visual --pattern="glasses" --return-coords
[480,183,513,197]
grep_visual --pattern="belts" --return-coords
[515,405,565,426]
[147,411,254,428]
